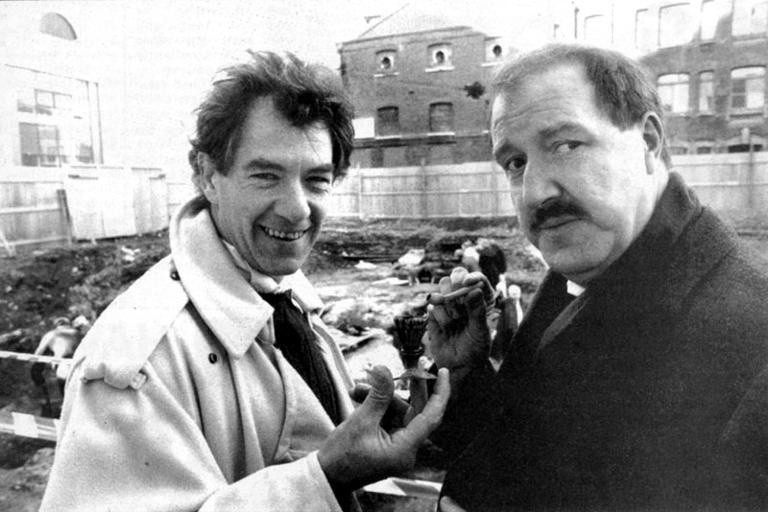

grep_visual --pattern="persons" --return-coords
[414,235,532,370]
[30,317,81,400]
[426,42,767,512]
[41,51,454,510]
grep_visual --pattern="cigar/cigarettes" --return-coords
[425,279,488,310]
